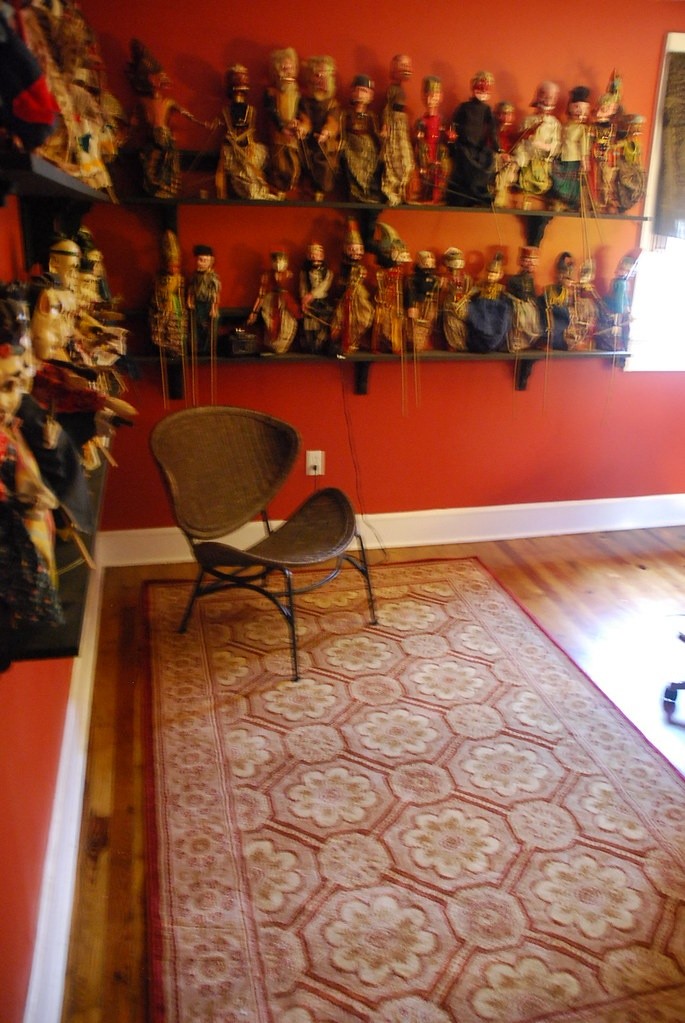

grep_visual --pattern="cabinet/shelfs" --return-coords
[125,198,649,398]
[0,154,112,672]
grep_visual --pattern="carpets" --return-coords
[137,554,685,1023]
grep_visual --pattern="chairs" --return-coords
[146,405,380,682]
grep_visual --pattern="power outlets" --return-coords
[306,451,325,475]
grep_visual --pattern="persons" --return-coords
[0,0,222,633]
[246,222,645,352]
[198,49,645,214]
